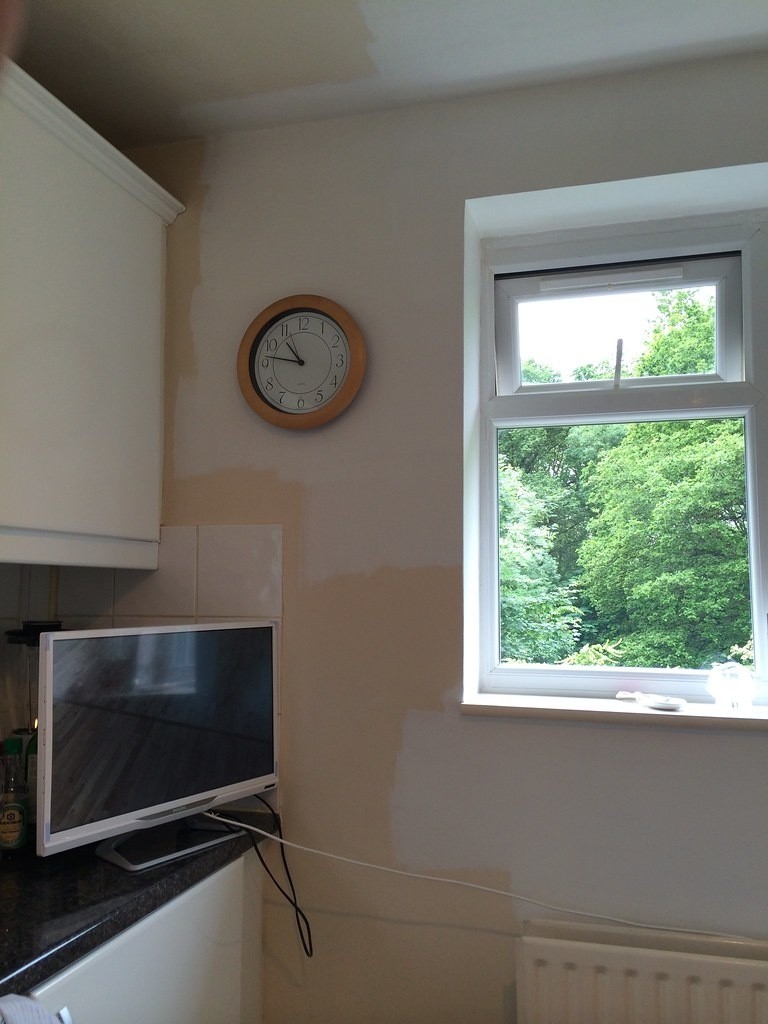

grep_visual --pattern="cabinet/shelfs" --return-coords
[0,56,184,571]
[28,851,244,1024]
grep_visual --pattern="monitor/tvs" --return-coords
[36,619,279,875]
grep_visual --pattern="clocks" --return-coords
[236,294,367,430]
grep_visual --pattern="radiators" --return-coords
[514,921,768,1024]
